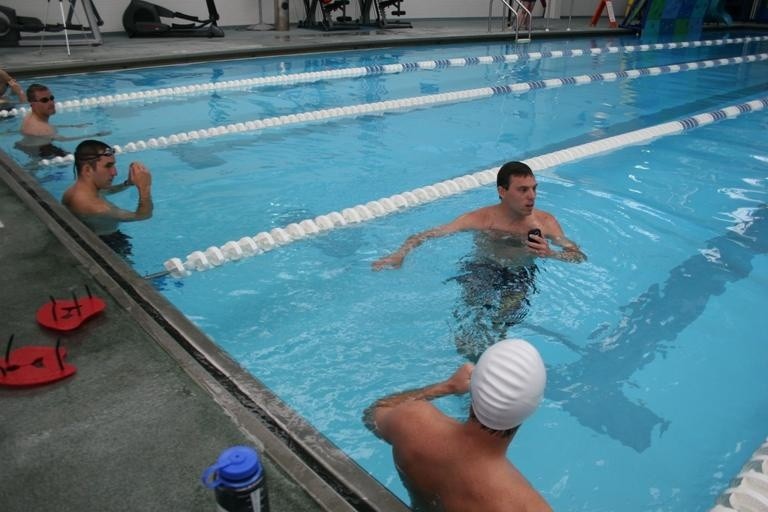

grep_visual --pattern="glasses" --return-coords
[34,95,54,102]
[75,147,116,161]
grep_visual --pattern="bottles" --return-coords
[199,445,271,512]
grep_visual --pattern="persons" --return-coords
[0,69,27,104]
[21,83,113,145]
[62,140,153,235]
[362,338,553,512]
[520,0,546,25]
[370,160,587,365]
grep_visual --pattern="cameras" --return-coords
[527,228,542,244]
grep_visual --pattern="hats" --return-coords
[469,338,546,431]
